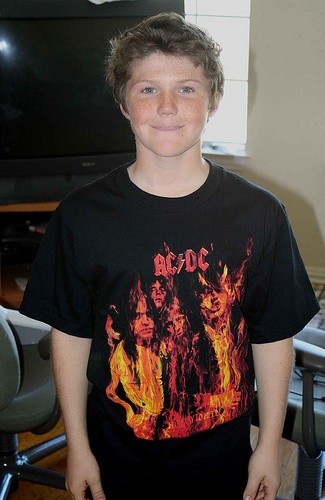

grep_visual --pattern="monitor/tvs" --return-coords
[0,0,184,201]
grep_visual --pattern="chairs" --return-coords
[0,304,88,500]
[250,327,324,498]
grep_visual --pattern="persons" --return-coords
[19,9,320,500]
